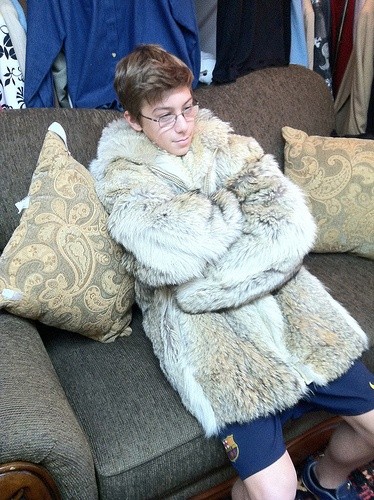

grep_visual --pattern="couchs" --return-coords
[0,65,374,500]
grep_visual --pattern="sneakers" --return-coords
[301,460,362,500]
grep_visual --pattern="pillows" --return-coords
[0,120,135,343]
[281,126,374,261]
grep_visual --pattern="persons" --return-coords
[89,44,374,500]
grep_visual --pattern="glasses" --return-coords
[140,95,201,127]
